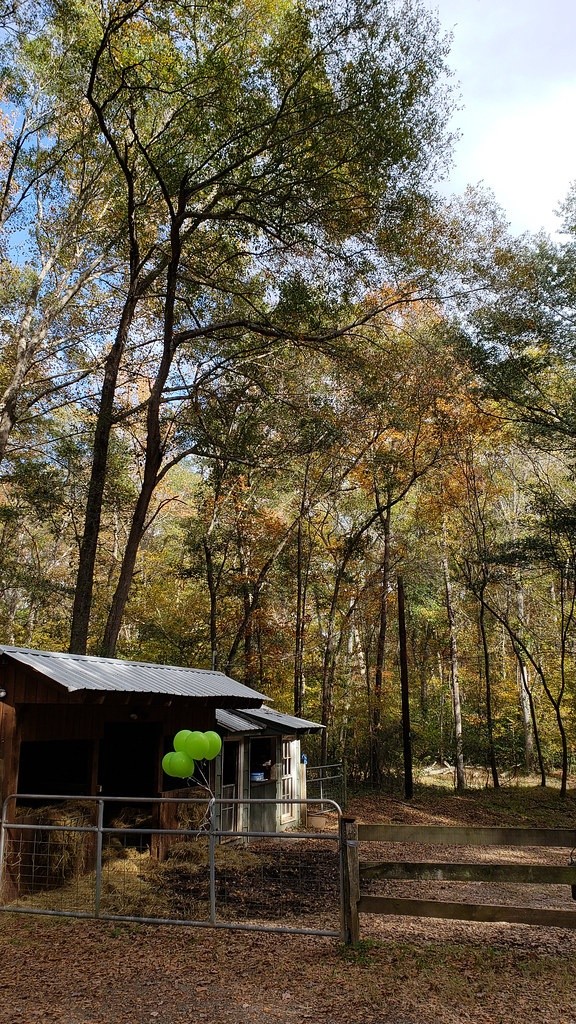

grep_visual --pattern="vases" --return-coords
[308,813,324,828]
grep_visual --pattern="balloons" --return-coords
[162,752,178,777]
[174,730,191,752]
[184,731,209,761]
[169,753,194,778]
[203,731,221,761]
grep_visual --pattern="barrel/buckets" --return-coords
[251,772,264,781]
